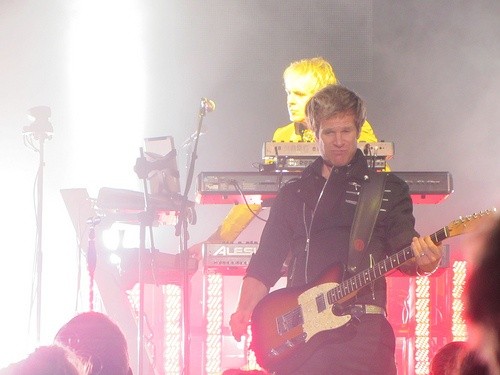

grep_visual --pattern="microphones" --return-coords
[201,98,215,112]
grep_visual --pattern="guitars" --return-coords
[250,206,496,375]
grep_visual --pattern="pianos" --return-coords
[197,170,453,207]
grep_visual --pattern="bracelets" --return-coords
[415,257,442,278]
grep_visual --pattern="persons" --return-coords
[0,312,130,375]
[228,85,442,375]
[183,57,392,265]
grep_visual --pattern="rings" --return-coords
[419,253,425,258]
